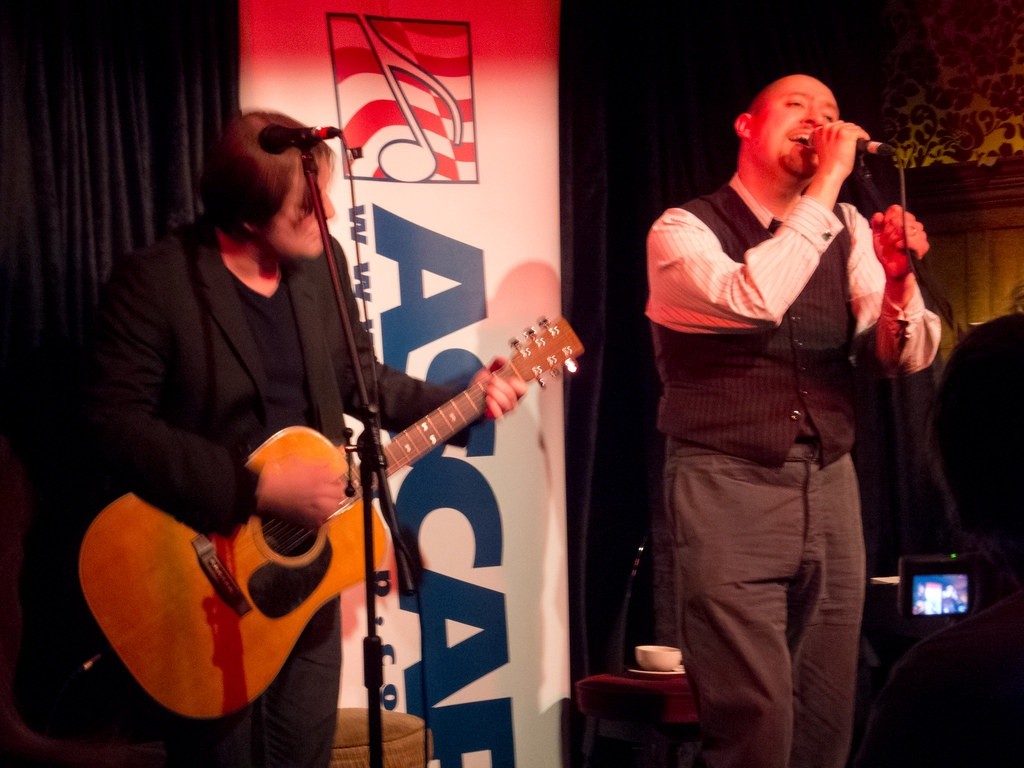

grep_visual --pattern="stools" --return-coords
[329,708,434,768]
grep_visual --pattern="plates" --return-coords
[629,665,687,678]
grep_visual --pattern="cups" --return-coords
[635,646,684,670]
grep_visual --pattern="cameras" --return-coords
[897,555,998,622]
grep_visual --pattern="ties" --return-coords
[768,217,782,235]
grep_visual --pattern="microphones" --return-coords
[259,124,342,155]
[808,130,897,155]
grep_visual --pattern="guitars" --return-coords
[64,314,588,729]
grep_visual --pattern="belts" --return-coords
[678,440,814,463]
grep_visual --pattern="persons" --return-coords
[641,73,943,767]
[855,309,1024,768]
[80,107,526,768]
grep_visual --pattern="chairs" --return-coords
[574,536,699,768]
